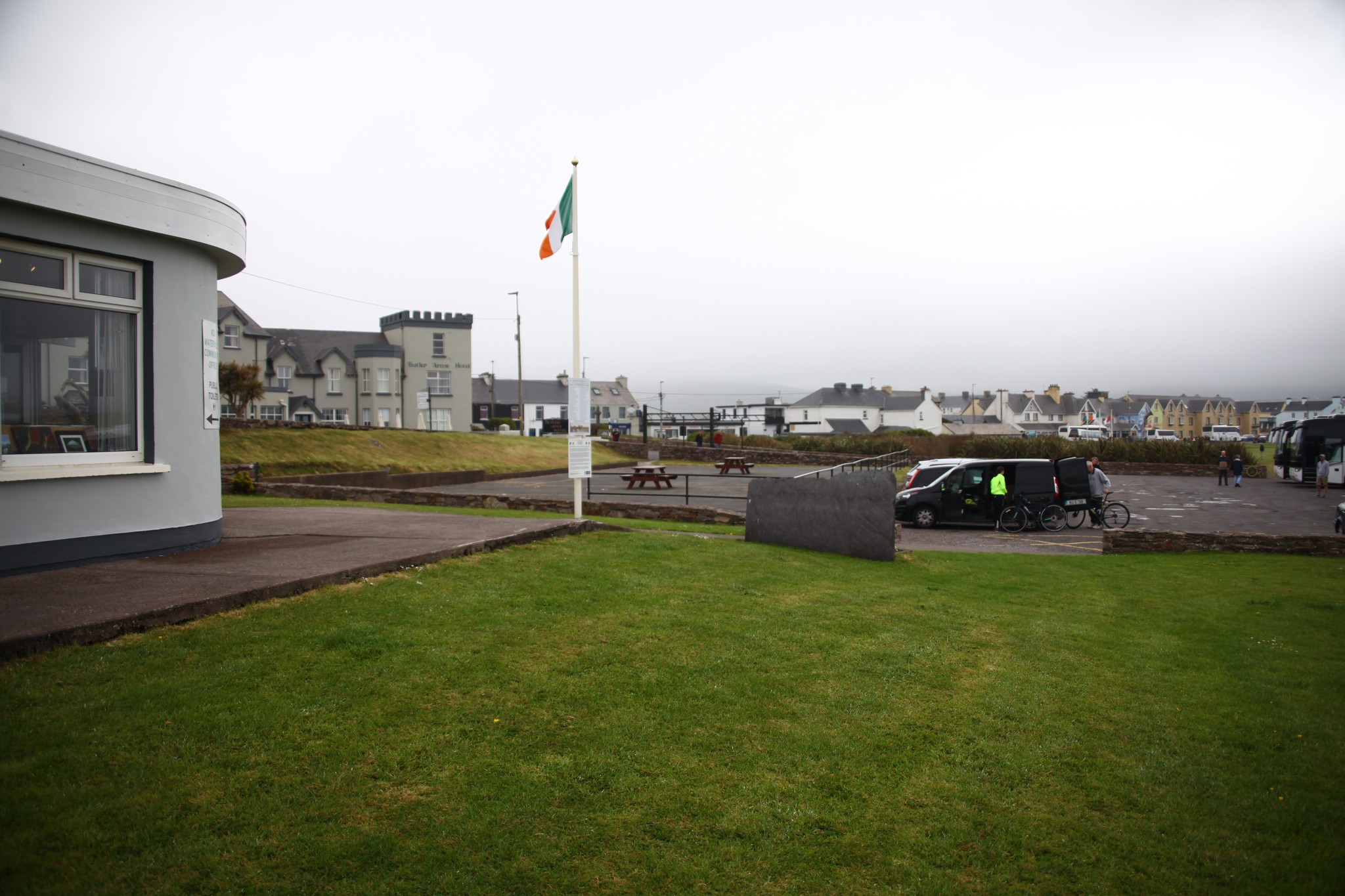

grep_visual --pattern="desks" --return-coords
[720,457,750,475]
[627,465,672,490]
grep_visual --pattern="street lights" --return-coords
[659,380,665,439]
[507,291,525,436]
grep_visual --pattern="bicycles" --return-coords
[1000,491,1068,533]
[1066,491,1131,530]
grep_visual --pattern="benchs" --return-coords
[715,463,755,469]
[620,474,678,480]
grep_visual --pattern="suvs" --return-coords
[895,457,1091,530]
[904,458,985,488]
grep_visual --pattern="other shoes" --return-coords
[1234,483,1241,487]
[994,528,1005,532]
[1314,494,1325,498]
[1087,523,1101,529]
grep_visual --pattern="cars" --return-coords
[1241,432,1268,441]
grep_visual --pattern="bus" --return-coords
[1287,414,1345,488]
[1269,419,1301,479]
[1058,424,1112,441]
[1204,425,1243,441]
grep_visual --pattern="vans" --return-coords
[1146,429,1180,441]
[1069,429,1108,442]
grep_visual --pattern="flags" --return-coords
[538,174,573,260]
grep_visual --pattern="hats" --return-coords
[1320,454,1325,457]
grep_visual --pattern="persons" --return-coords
[612,427,621,441]
[1314,454,1330,498]
[696,431,703,447]
[1218,450,1229,486]
[1230,455,1243,487]
[1259,442,1265,460]
[990,467,1012,526]
[714,431,722,448]
[1091,456,1103,472]
[1086,461,1111,530]
